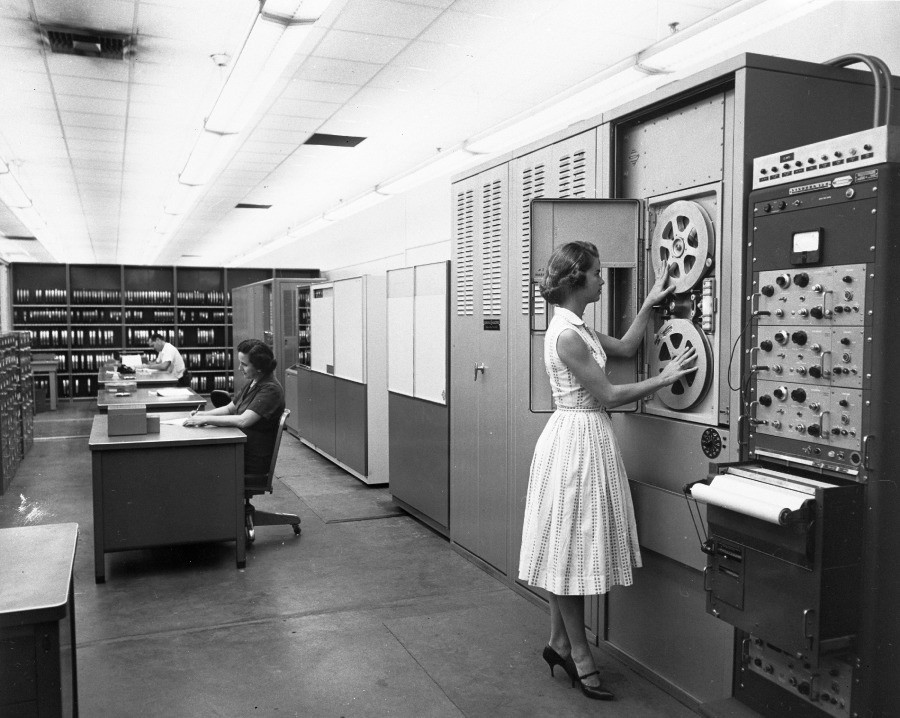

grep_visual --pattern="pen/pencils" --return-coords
[196,404,201,413]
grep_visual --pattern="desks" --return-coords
[0,522,78,718]
[31,360,59,410]
[88,366,248,584]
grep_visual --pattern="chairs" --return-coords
[210,390,231,408]
[243,409,302,544]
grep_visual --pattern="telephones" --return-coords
[117,364,136,374]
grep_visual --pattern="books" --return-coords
[298,292,310,366]
[13,288,234,397]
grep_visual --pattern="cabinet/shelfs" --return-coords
[231,278,327,392]
[12,305,234,403]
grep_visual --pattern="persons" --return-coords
[518,240,698,701]
[183,338,286,485]
[146,333,191,387]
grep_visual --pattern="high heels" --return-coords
[566,656,616,699]
[542,642,570,677]
